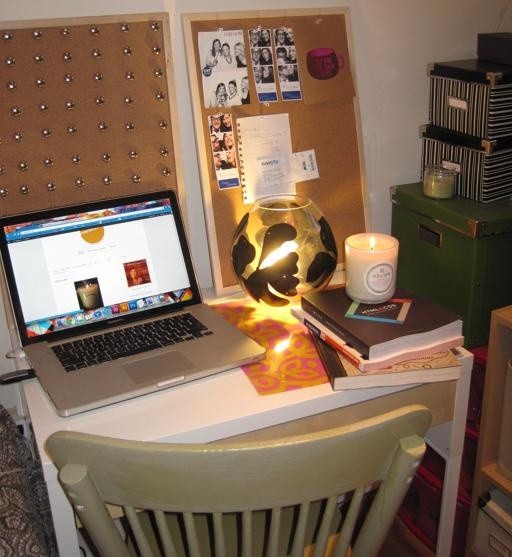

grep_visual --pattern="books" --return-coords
[290,283,465,392]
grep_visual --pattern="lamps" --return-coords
[228,190,341,318]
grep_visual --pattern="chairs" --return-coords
[38,401,439,556]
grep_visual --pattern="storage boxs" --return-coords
[461,342,493,443]
[474,31,511,66]
[424,55,512,142]
[416,424,476,508]
[395,457,471,557]
[415,120,511,204]
[385,175,511,354]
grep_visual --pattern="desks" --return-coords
[7,260,478,556]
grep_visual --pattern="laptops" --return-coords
[1,189,267,418]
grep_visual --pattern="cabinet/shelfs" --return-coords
[465,298,511,556]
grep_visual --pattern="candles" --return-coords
[422,160,455,202]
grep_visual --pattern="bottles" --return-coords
[231,194,339,308]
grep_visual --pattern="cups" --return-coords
[423,164,457,200]
[306,46,345,78]
[78,283,100,308]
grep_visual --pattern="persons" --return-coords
[205,27,298,171]
[126,268,142,286]
